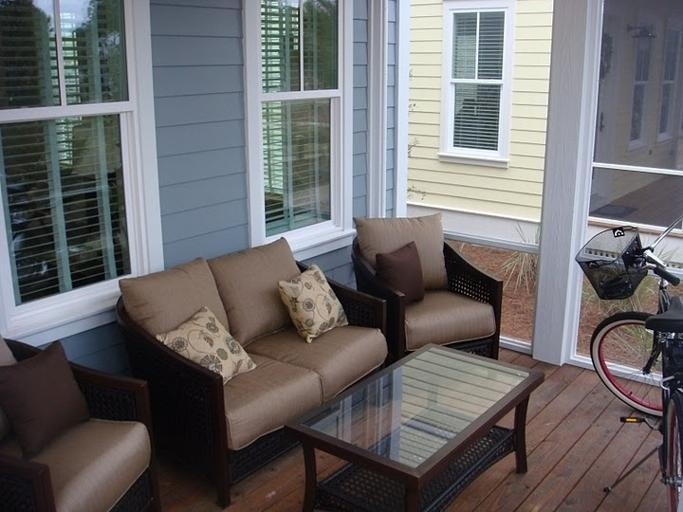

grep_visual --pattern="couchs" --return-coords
[353,234,502,362]
[110,261,388,506]
[1,339,153,512]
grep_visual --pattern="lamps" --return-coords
[627,24,656,52]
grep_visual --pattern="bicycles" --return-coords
[575,214,682,511]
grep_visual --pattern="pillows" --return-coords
[376,243,425,306]
[279,266,348,342]
[357,213,448,292]
[209,238,300,348]
[0,339,89,460]
[119,258,232,335]
[156,308,256,384]
